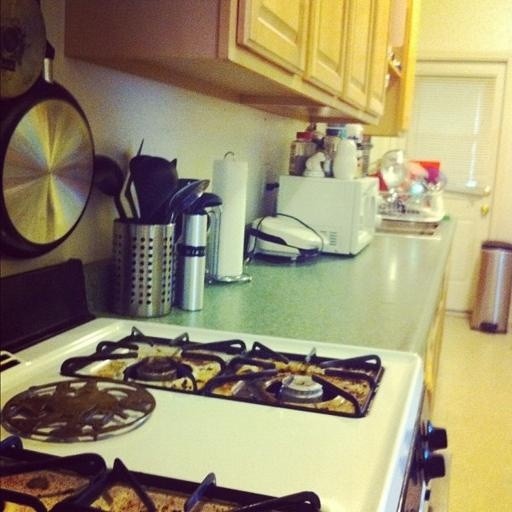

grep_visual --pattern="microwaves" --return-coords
[274,175,380,257]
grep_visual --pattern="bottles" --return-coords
[288,125,366,178]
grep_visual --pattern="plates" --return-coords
[384,213,446,233]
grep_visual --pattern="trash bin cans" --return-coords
[469,239,512,335]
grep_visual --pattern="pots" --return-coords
[0,37,95,258]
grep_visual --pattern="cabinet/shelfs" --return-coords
[66,0,343,112]
[261,2,389,123]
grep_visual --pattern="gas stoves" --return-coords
[0,317,446,512]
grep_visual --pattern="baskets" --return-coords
[379,188,445,218]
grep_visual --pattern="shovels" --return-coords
[124,136,145,216]
